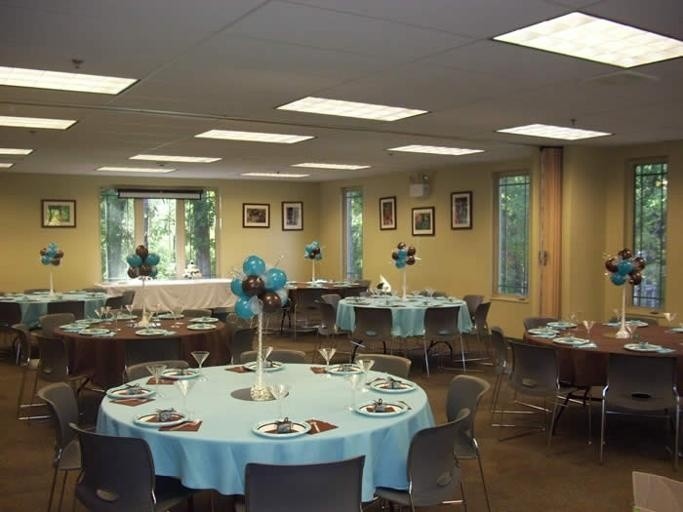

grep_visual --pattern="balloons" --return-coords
[304,240,322,262]
[392,240,417,270]
[231,256,290,322]
[40,241,65,267]
[605,249,647,287]
[127,244,162,278]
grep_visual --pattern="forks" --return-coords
[310,417,320,433]
[115,398,148,402]
[168,418,200,431]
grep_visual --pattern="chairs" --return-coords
[10,307,259,447]
[0,286,136,368]
[314,290,492,380]
[280,279,372,341]
[487,313,683,465]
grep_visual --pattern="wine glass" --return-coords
[146,365,167,398]
[95,303,184,331]
[319,348,336,372]
[611,308,622,326]
[255,346,273,361]
[268,385,291,419]
[346,287,463,307]
[356,359,375,385]
[344,373,366,412]
[191,351,209,376]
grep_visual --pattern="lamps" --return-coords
[422,174,429,182]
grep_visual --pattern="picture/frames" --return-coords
[243,203,271,228]
[379,196,397,230]
[41,198,76,228]
[411,206,435,236]
[450,191,472,230]
[281,201,304,231]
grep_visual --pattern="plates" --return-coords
[528,312,682,352]
[253,418,312,438]
[105,386,156,398]
[326,363,361,376]
[285,279,360,288]
[371,379,416,393]
[0,289,106,302]
[61,314,219,336]
[160,368,200,379]
[245,361,285,371]
[133,413,188,427]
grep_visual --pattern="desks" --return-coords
[92,277,242,313]
